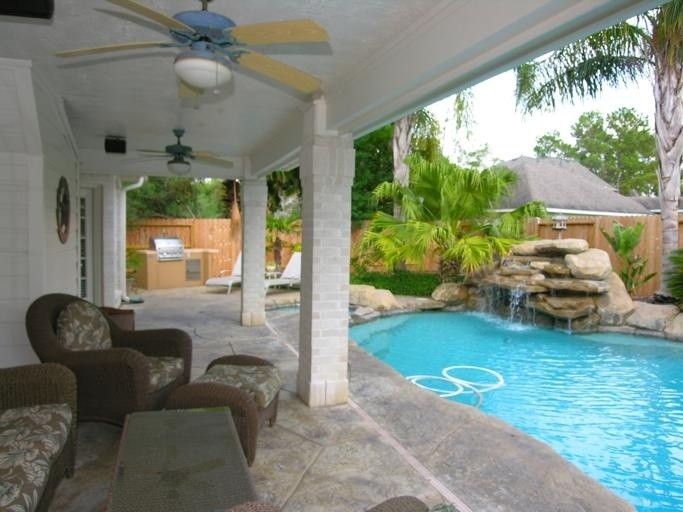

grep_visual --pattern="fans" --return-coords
[53,0,332,95]
[132,128,234,169]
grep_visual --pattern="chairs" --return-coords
[24,293,194,427]
[205,250,301,295]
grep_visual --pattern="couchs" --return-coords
[0,362,79,512]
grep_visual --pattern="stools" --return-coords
[164,355,281,467]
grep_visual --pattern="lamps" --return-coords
[164,155,191,176]
[173,47,235,92]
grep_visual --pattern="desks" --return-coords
[139,249,219,291]
